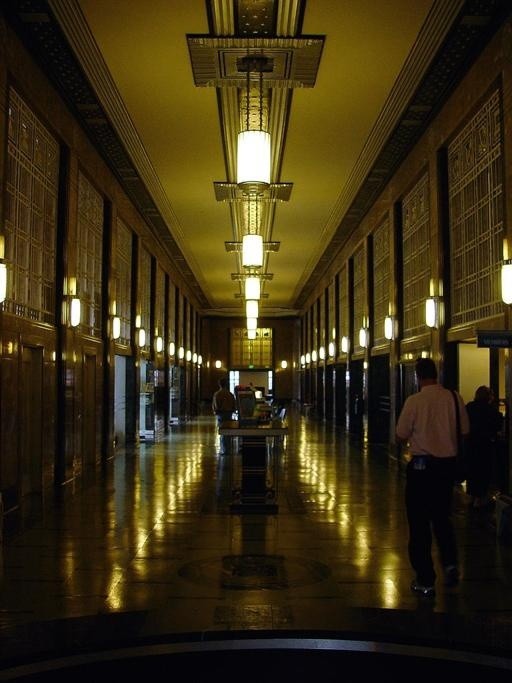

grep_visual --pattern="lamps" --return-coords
[0,264,7,305]
[241,197,263,341]
[235,51,276,198]
[70,298,203,366]
[501,260,512,306]
[300,296,437,365]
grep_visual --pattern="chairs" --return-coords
[275,407,288,456]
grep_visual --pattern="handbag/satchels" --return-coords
[458,444,474,482]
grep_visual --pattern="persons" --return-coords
[394,358,470,595]
[465,384,511,514]
[233,383,249,399]
[213,377,237,457]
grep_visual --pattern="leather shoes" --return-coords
[443,569,459,588]
[411,581,436,597]
